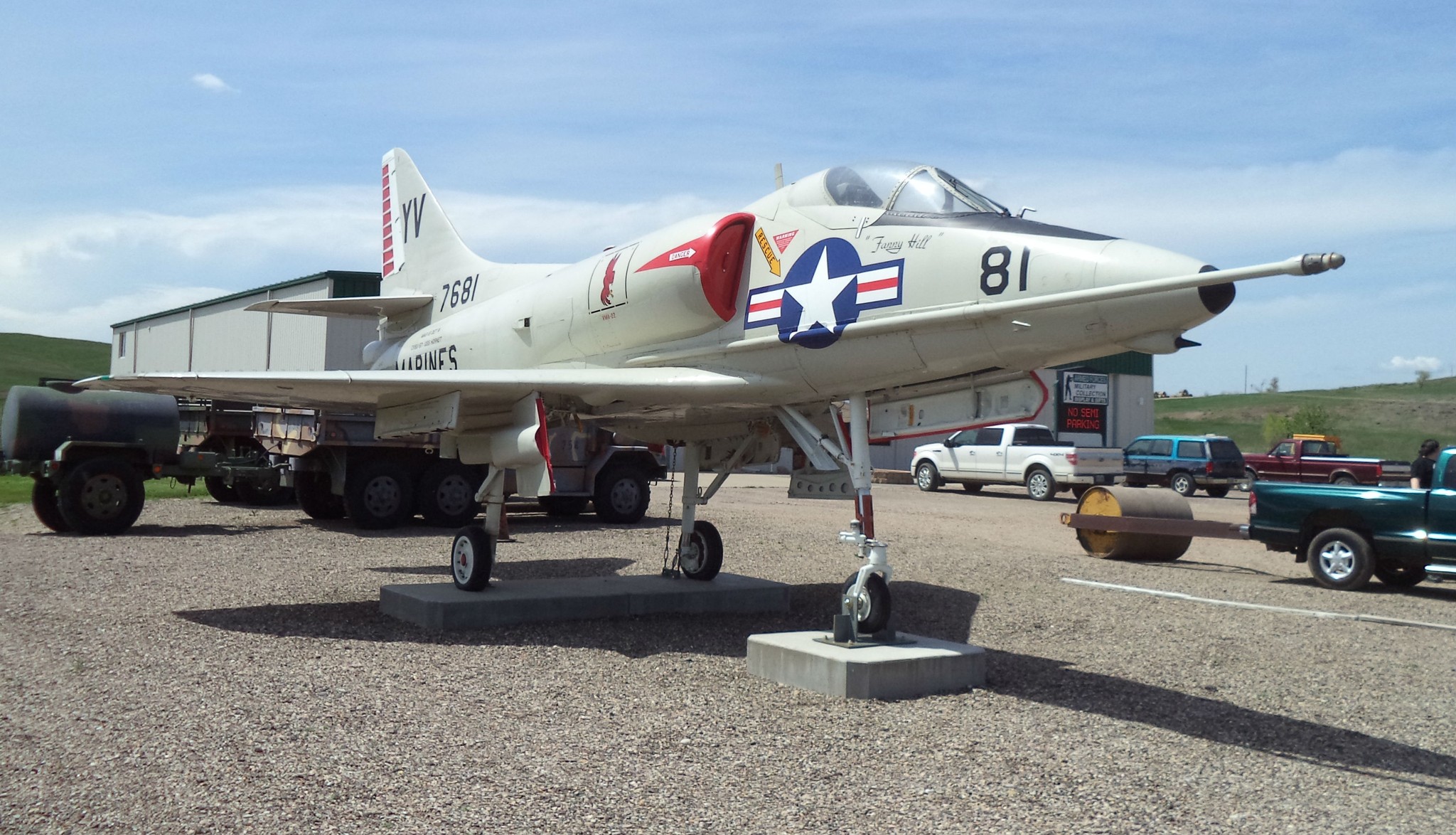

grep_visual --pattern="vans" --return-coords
[1122,434,1250,498]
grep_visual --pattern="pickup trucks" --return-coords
[911,423,1124,501]
[1239,447,1456,590]
[1236,439,1413,492]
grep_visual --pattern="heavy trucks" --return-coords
[0,385,667,535]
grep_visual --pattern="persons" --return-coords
[1410,439,1441,490]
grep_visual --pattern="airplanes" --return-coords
[71,146,1347,635]
